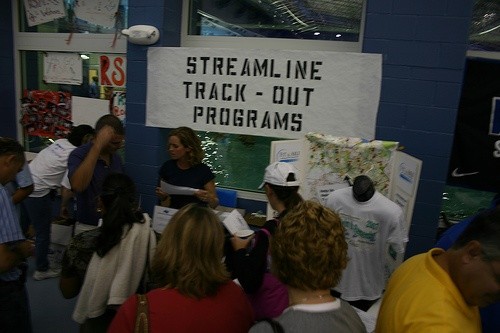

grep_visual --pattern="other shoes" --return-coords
[33,268,59,280]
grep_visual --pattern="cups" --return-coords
[237,231,254,248]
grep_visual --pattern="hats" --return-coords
[257,162,300,190]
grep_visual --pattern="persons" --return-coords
[151,126,221,212]
[8,159,34,221]
[19,123,96,281]
[0,136,36,333]
[324,175,410,313]
[106,203,256,333]
[372,208,500,332]
[228,162,306,319]
[246,201,368,333]
[64,115,160,332]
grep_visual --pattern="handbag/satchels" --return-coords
[251,216,289,322]
